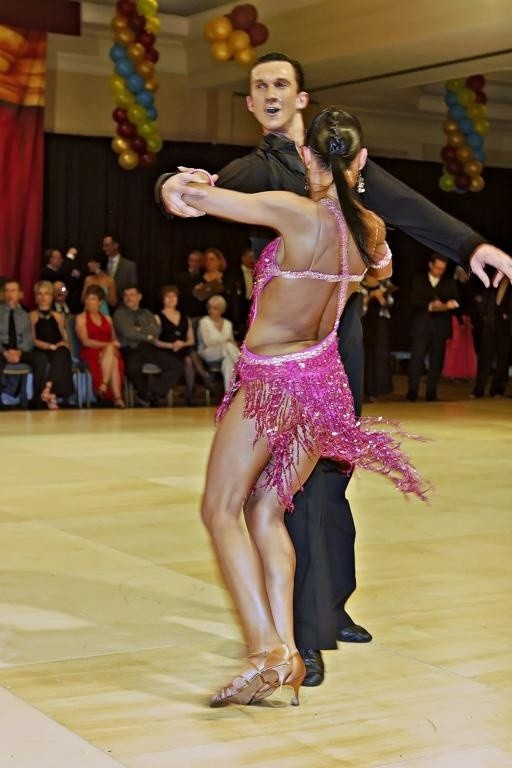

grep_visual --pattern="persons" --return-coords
[1,231,259,409]
[361,244,512,401]
[152,53,512,687]
[179,107,398,711]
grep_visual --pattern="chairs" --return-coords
[3,314,221,407]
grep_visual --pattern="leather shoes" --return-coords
[298,646,324,686]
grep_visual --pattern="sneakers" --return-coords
[468,385,484,399]
[488,386,501,400]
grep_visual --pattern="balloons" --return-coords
[203,3,269,66]
[437,72,489,194]
[107,0,163,171]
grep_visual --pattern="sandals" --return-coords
[41,386,59,410]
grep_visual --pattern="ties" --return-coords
[9,309,18,350]
[110,260,116,273]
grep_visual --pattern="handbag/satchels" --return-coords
[440,335,477,380]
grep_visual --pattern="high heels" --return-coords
[249,652,306,706]
[336,624,372,642]
[210,643,293,706]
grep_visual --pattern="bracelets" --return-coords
[189,168,214,186]
